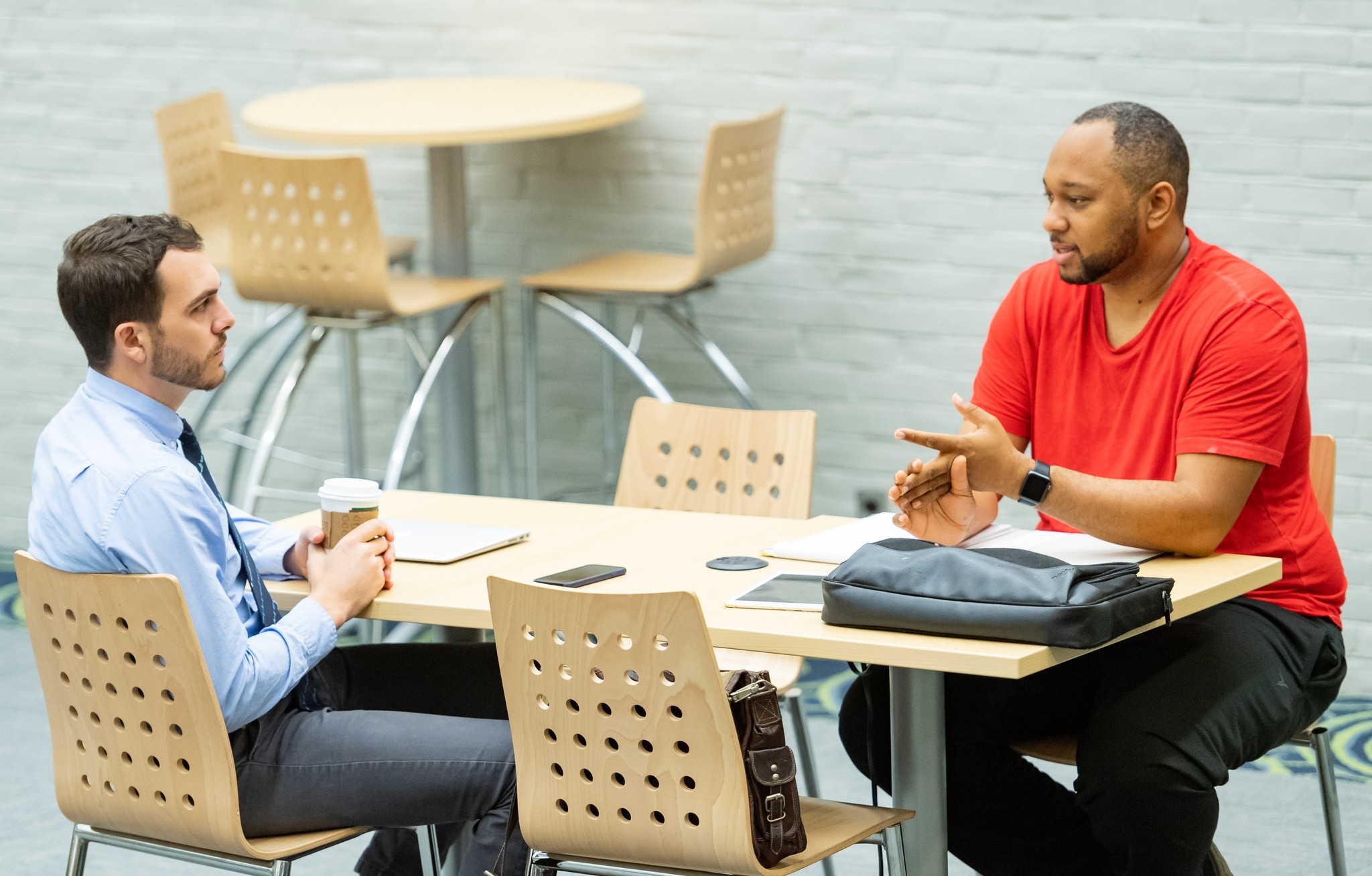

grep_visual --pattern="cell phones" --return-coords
[533,566,626,588]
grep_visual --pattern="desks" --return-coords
[230,490,1281,876]
[242,74,644,496]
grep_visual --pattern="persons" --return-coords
[838,102,1347,876]
[28,212,529,876]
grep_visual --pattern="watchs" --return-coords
[1018,460,1051,507]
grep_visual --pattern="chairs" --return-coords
[983,433,1347,876]
[610,396,832,875]
[516,106,787,501]
[153,87,419,513]
[13,549,446,876]
[217,141,508,516]
[485,575,915,876]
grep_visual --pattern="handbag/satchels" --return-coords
[721,667,808,863]
[820,537,1177,646]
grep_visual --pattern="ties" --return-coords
[179,416,328,712]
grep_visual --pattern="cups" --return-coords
[317,478,386,554]
